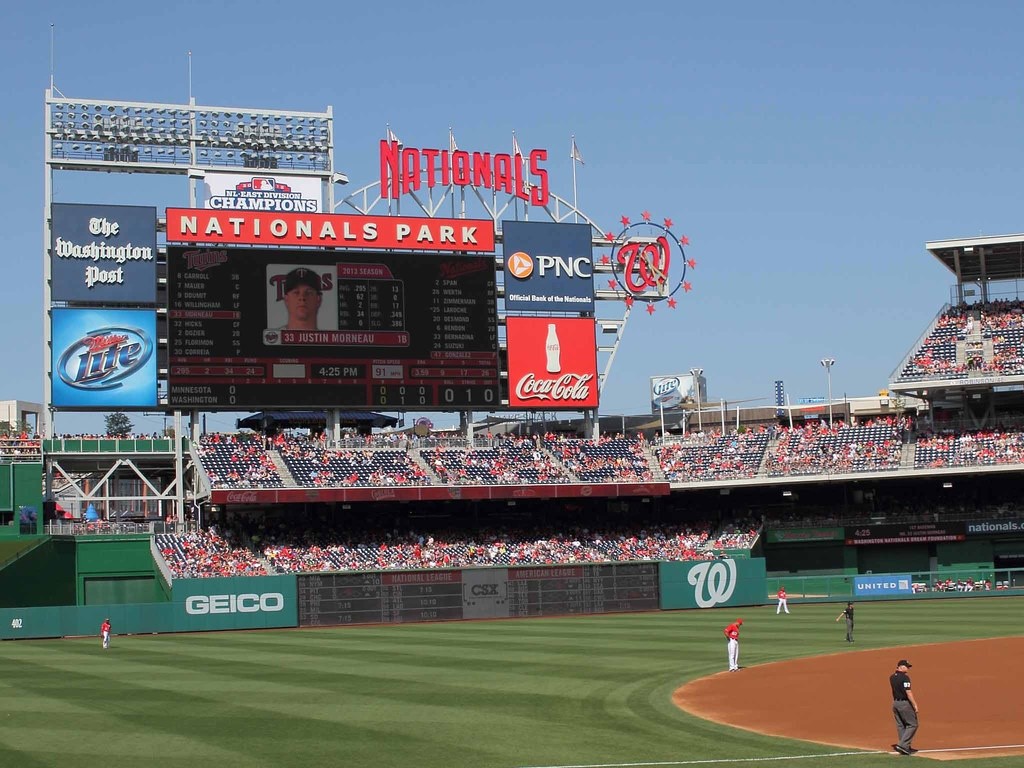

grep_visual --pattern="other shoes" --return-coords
[896,745,910,755]
[909,748,919,753]
[850,640,854,644]
[734,669,740,672]
[730,669,735,672]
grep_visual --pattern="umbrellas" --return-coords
[86,503,98,522]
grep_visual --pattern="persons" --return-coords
[836,602,854,643]
[74,513,111,534]
[651,410,1024,481]
[0,432,171,463]
[912,578,992,592]
[276,268,323,331]
[776,586,790,614]
[767,494,1024,528]
[723,618,743,674]
[200,427,650,491]
[101,618,112,649]
[156,503,767,581]
[906,295,1024,377]
[889,660,919,755]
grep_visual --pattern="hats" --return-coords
[898,659,912,667]
[736,619,742,625]
[284,268,321,294]
[847,601,853,605]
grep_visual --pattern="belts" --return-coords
[732,637,738,642]
[894,697,909,701]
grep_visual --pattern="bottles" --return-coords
[545,322,561,373]
